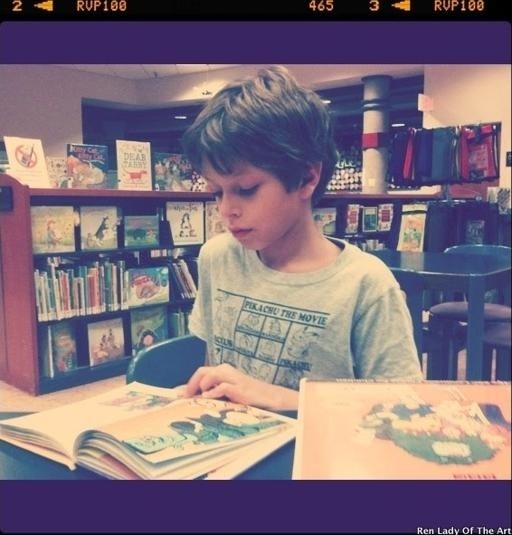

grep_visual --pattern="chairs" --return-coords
[442,245,510,380]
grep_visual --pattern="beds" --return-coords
[359,250,512,383]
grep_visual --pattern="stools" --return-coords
[447,318,512,381]
[428,300,512,383]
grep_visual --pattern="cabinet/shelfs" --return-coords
[0,166,511,396]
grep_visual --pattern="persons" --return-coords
[175,63,423,412]
[0,380,297,480]
[311,207,342,235]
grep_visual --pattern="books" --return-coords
[378,204,394,232]
[124,215,161,250]
[30,207,75,252]
[87,318,125,367]
[171,308,192,339]
[166,201,205,245]
[117,140,152,191]
[129,266,170,306]
[167,257,198,299]
[152,152,211,192]
[34,256,129,323]
[80,205,118,251]
[46,156,66,187]
[396,205,430,252]
[130,305,169,357]
[292,378,512,480]
[51,323,78,373]
[343,238,390,252]
[205,200,231,242]
[346,205,360,233]
[362,207,378,233]
[66,142,108,189]
